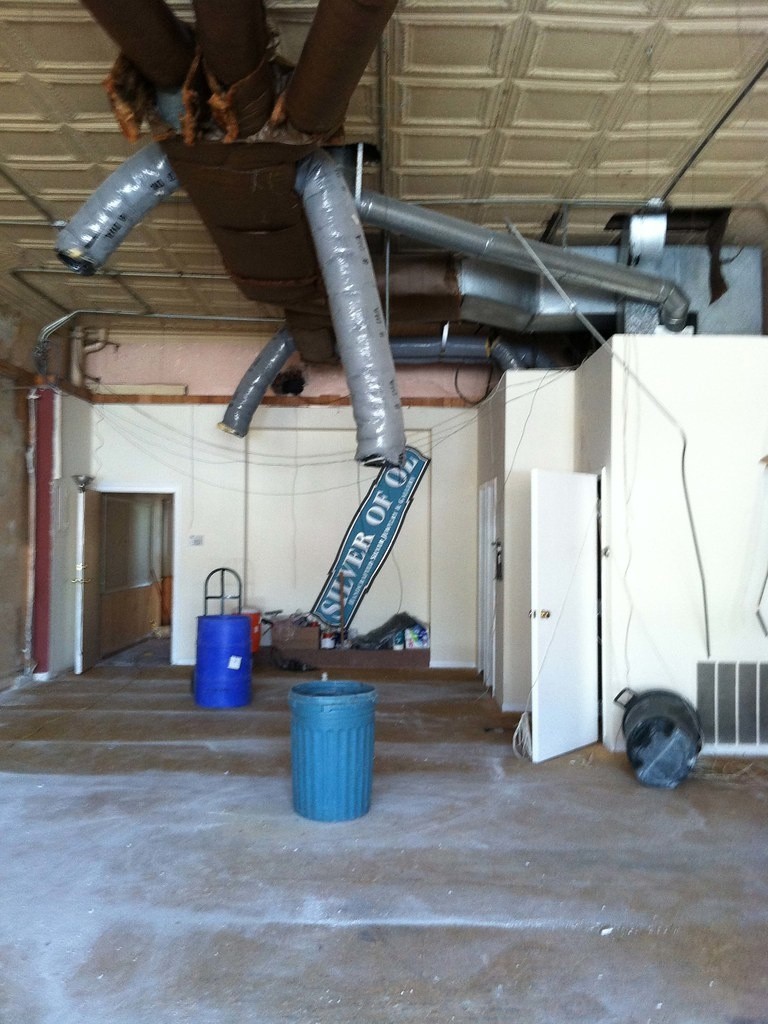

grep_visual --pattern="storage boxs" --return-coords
[270,626,320,650]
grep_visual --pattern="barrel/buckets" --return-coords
[194,614,253,708]
[233,608,260,653]
